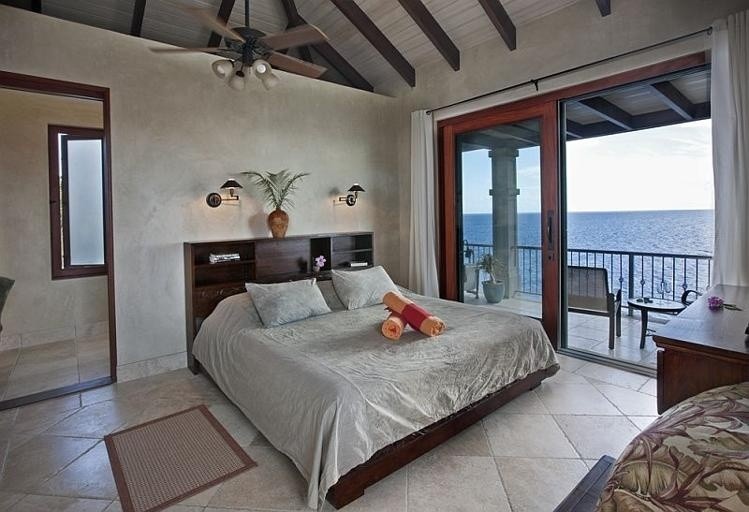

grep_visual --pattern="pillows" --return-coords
[244,264,446,340]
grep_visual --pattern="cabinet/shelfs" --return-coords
[185,231,376,318]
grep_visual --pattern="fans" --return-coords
[147,1,331,80]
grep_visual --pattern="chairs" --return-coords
[566,266,623,350]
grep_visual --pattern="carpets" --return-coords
[554,455,618,511]
[103,402,259,511]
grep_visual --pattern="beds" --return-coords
[195,279,560,509]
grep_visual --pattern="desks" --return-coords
[628,296,686,350]
[651,284,749,417]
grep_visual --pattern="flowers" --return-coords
[706,293,743,314]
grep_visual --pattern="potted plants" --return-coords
[477,252,509,304]
[238,167,311,240]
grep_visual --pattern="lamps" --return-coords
[211,43,281,91]
[206,178,243,209]
[338,184,366,206]
